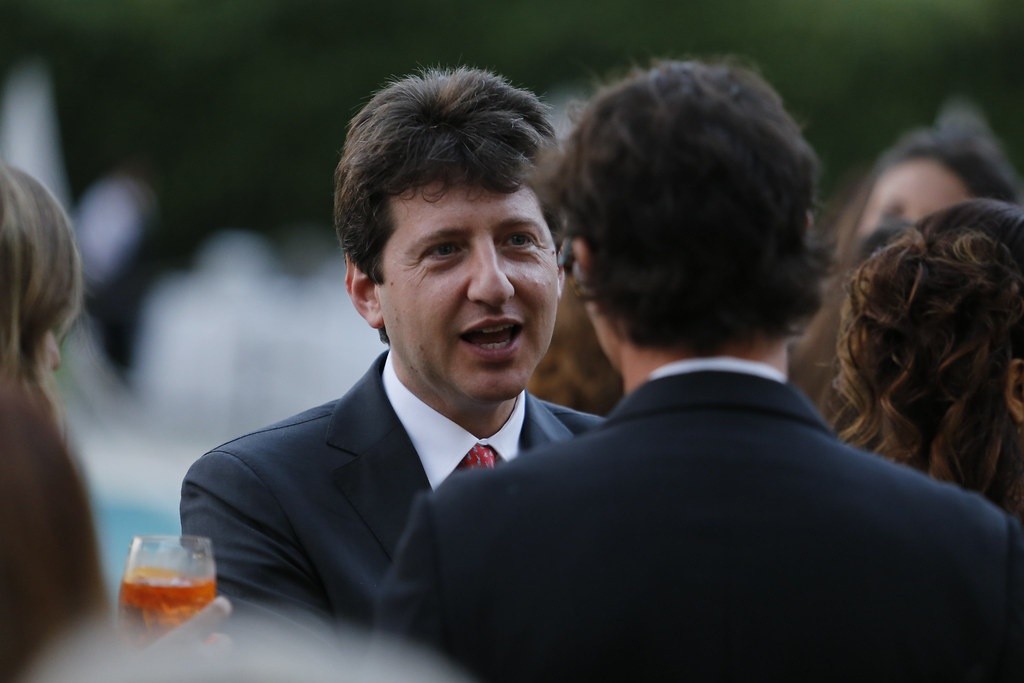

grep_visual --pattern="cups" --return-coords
[117,536,217,652]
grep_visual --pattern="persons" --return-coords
[179,65,612,651]
[784,109,1024,519]
[381,60,1024,683]
[0,160,235,683]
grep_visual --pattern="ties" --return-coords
[459,442,497,471]
